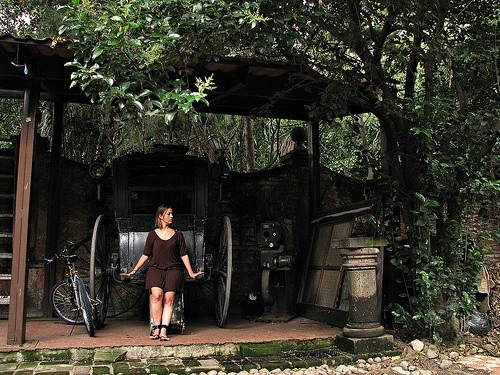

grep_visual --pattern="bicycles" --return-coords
[40,240,102,337]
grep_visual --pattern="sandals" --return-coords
[159,325,170,341]
[150,325,161,340]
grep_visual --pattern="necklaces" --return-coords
[160,228,169,237]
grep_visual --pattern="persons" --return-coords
[120,204,204,342]
